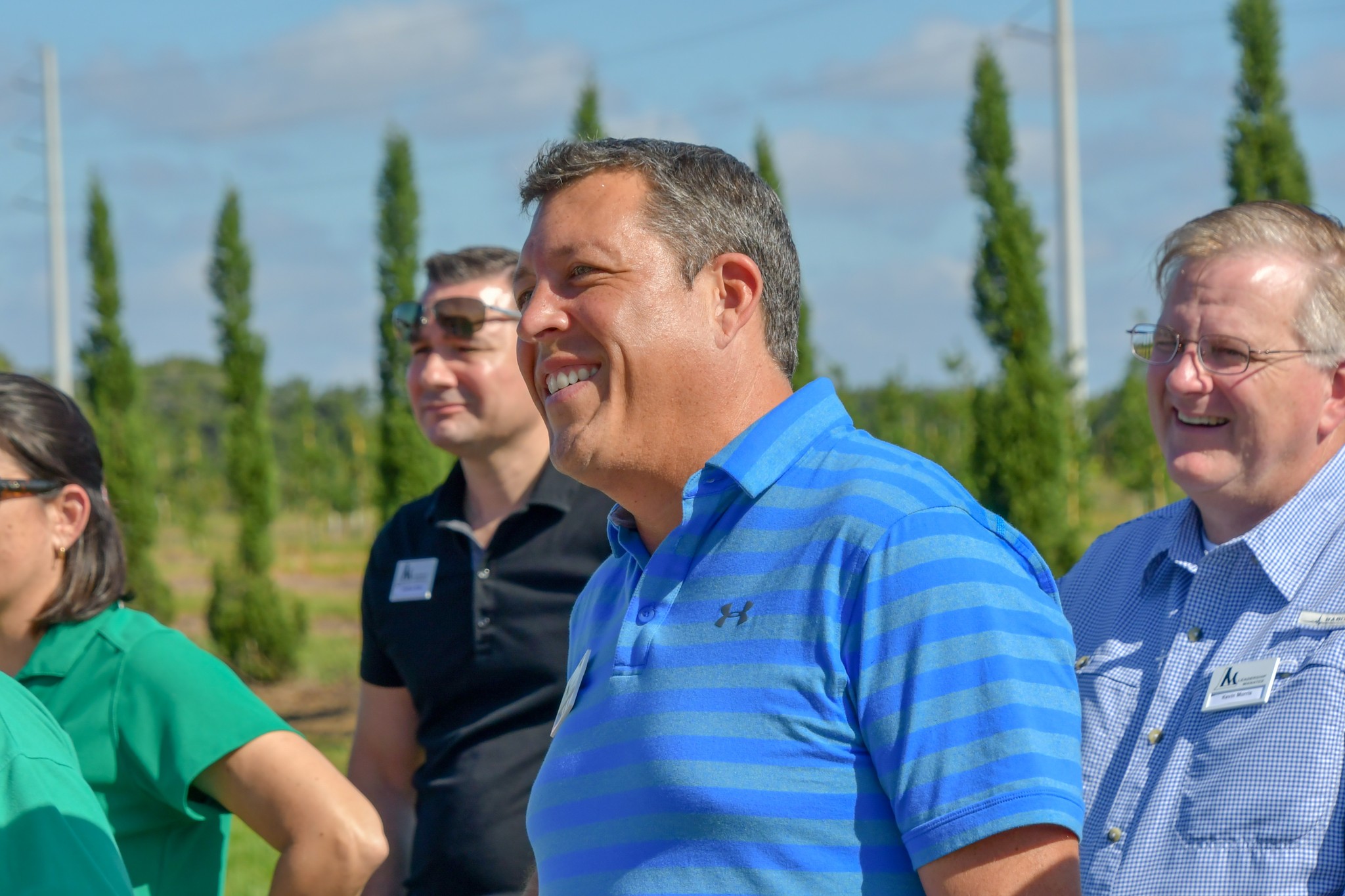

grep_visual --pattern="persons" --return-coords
[0,670,133,895]
[1058,200,1345,896]
[512,137,1086,895]
[349,249,617,895]
[0,370,389,895]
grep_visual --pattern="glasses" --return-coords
[1127,322,1341,375]
[391,296,521,343]
